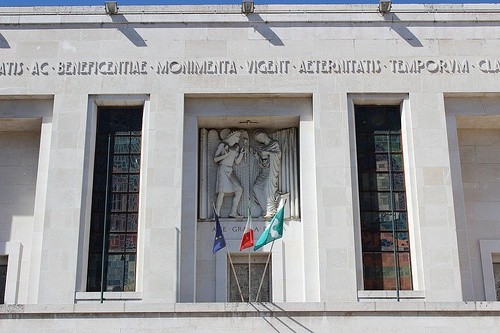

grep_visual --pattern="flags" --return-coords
[211,200,227,254]
[239,200,255,251]
[253,196,284,251]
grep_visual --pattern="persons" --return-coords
[212,130,244,220]
[249,130,282,220]
